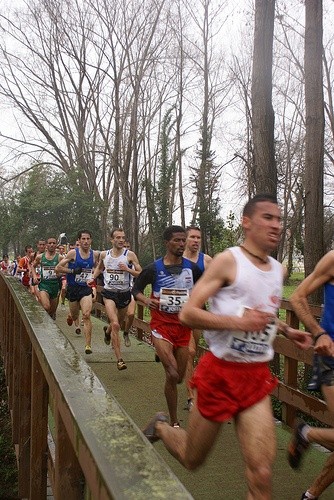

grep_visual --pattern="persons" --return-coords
[288,247,334,500]
[87,229,144,371]
[55,240,82,310]
[31,238,64,314]
[55,229,97,354]
[180,228,215,413]
[140,194,312,500]
[108,239,135,347]
[17,238,48,304]
[132,226,207,428]
[0,250,24,283]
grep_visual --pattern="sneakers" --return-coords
[85,344,92,354]
[123,334,130,347]
[116,361,127,370]
[143,410,167,444]
[288,420,309,469]
[104,326,110,345]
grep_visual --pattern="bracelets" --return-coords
[92,278,96,282]
[70,269,73,274]
[27,269,29,272]
[282,326,292,338]
[314,332,328,344]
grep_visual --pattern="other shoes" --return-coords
[61,303,66,311]
[75,328,80,334]
[67,313,73,326]
[183,398,192,411]
[171,423,180,428]
[81,320,84,326]
[155,353,160,362]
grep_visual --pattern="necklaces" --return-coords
[238,245,269,265]
